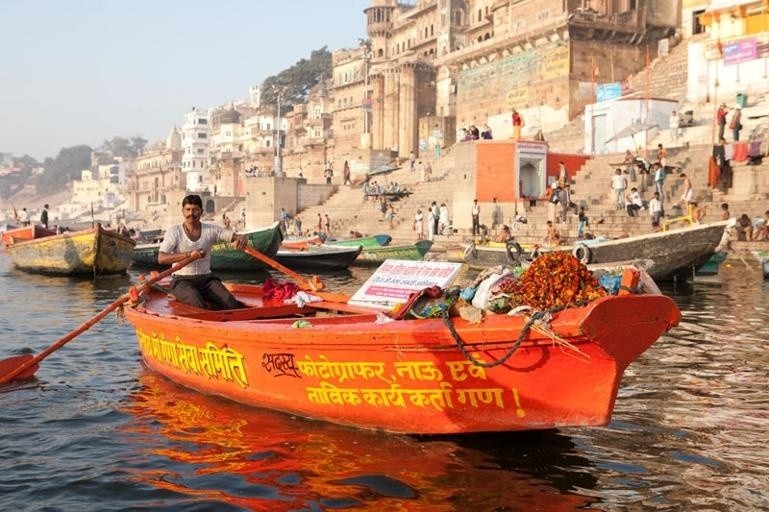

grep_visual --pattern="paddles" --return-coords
[1,248,206,385]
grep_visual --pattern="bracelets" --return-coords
[185,251,193,258]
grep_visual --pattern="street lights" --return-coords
[357,35,375,151]
[271,76,290,173]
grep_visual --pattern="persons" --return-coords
[40,205,50,229]
[105,223,143,238]
[156,195,248,310]
[63,227,71,233]
[16,208,30,226]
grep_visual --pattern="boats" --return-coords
[445,238,546,262]
[121,268,682,437]
[695,249,731,276]
[457,216,737,285]
[122,362,621,512]
